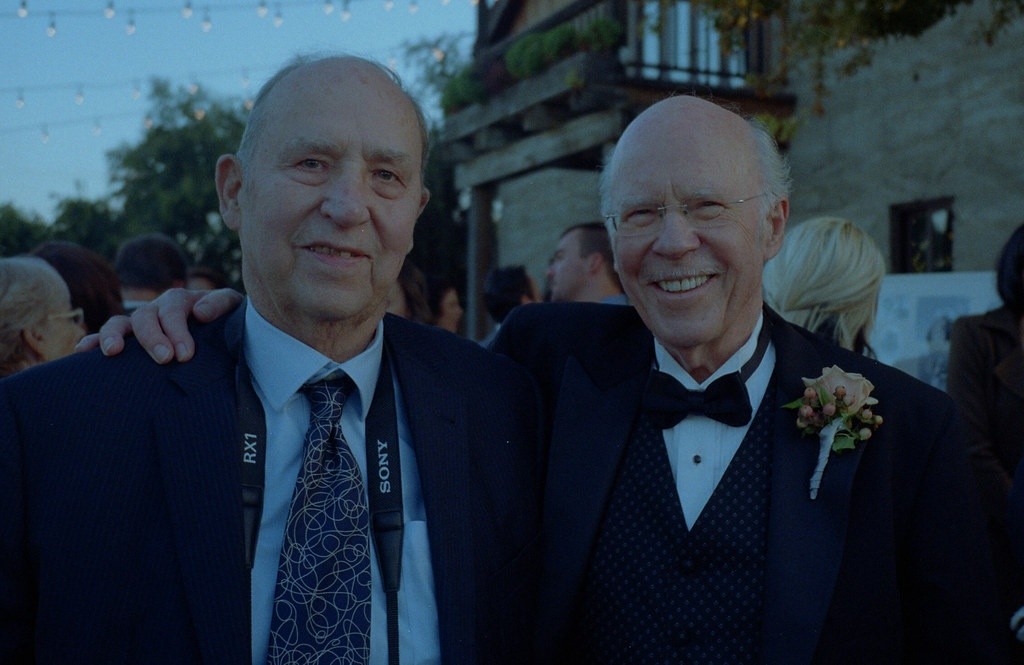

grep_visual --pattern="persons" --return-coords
[386,223,631,349]
[763,215,882,361]
[1,230,226,379]
[946,224,1024,665]
[0,55,541,665]
[74,94,1015,665]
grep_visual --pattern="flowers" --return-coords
[632,0,1024,118]
[780,366,882,498]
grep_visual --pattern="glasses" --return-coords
[46,307,84,326]
[602,193,764,237]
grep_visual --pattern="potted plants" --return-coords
[506,34,561,132]
[456,68,507,153]
[548,16,629,117]
[437,76,474,167]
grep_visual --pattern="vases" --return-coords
[472,53,512,99]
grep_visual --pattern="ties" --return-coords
[266,376,372,665]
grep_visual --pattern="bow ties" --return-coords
[644,313,772,430]
